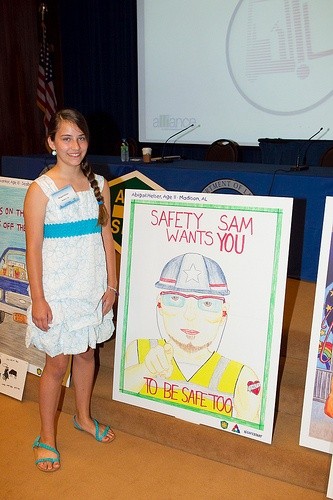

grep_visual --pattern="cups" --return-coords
[142,147,152,163]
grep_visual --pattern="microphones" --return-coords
[291,127,323,171]
[156,124,194,163]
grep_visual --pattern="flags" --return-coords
[34,21,60,125]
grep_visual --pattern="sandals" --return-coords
[32,433,61,473]
[73,414,115,443]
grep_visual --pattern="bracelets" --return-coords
[108,286,117,292]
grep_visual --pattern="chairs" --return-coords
[320,148,333,167]
[205,139,241,162]
[114,137,139,156]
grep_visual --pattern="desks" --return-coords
[0,153,333,283]
[258,139,333,165]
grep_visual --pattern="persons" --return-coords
[23,110,118,473]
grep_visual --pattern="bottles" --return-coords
[120,138,130,162]
